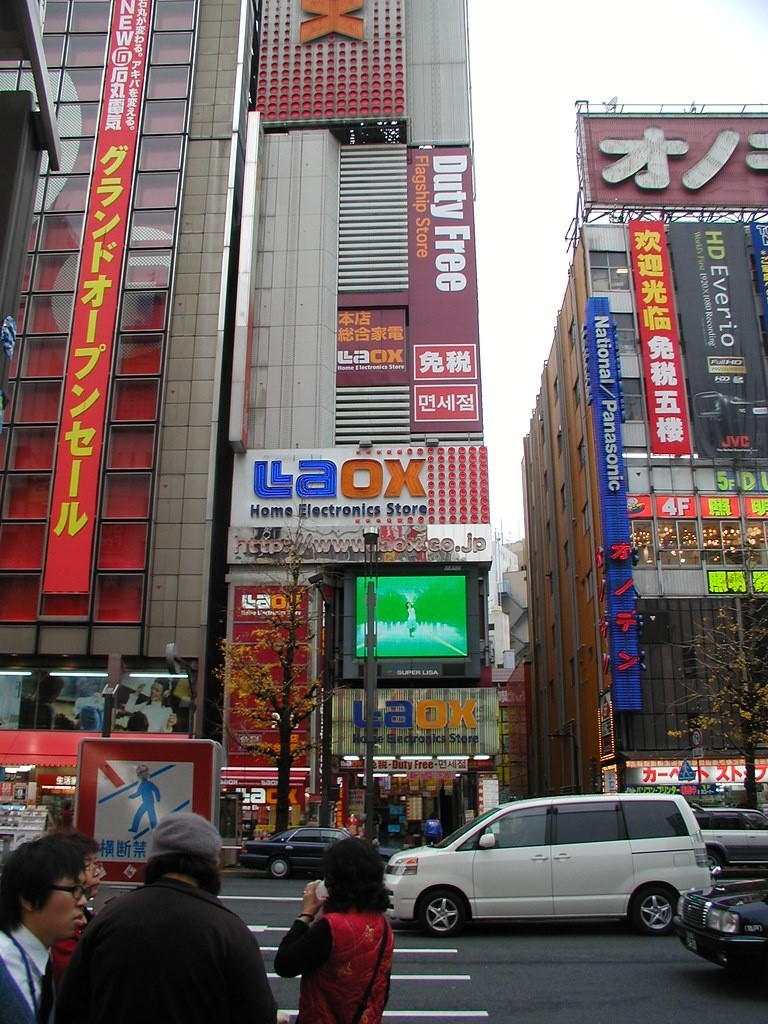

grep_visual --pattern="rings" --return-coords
[302,890,306,894]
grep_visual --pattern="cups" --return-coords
[314,880,329,901]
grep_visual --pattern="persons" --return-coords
[0,838,89,1024]
[406,602,417,637]
[51,834,101,990]
[423,811,443,845]
[19,677,104,730]
[372,836,380,848]
[124,678,178,734]
[346,814,358,835]
[55,812,280,1024]
[273,837,398,1024]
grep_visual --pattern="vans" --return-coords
[381,792,711,939]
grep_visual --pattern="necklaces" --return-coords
[7,933,39,1013]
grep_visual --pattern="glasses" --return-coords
[53,884,92,901]
[85,860,103,871]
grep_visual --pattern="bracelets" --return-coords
[298,914,315,921]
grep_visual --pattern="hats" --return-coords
[146,812,222,867]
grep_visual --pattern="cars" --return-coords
[669,875,768,983]
[695,808,768,875]
[236,828,403,880]
